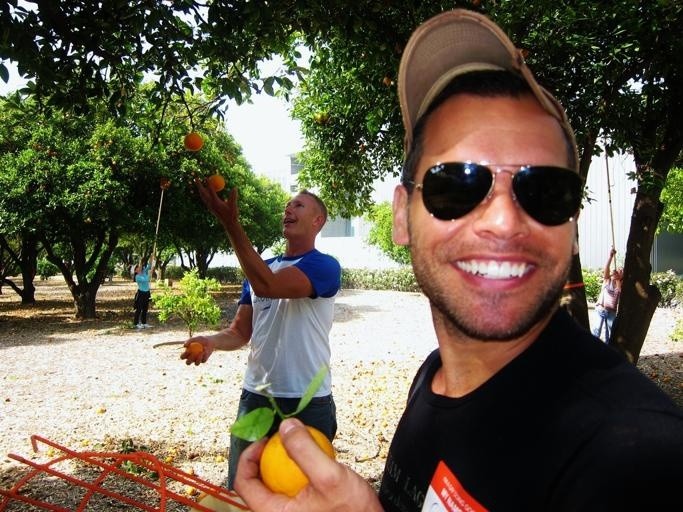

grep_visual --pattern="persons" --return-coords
[131,253,153,330]
[181,177,341,493]
[233,10,683,512]
[593,248,624,339]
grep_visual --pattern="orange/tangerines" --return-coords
[184,133,202,150]
[187,487,197,496]
[229,365,336,499]
[187,342,204,361]
[207,175,225,192]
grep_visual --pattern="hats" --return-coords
[130,263,138,280]
[397,10,580,175]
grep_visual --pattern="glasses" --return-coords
[405,161,584,226]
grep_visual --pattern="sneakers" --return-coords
[134,323,153,329]
